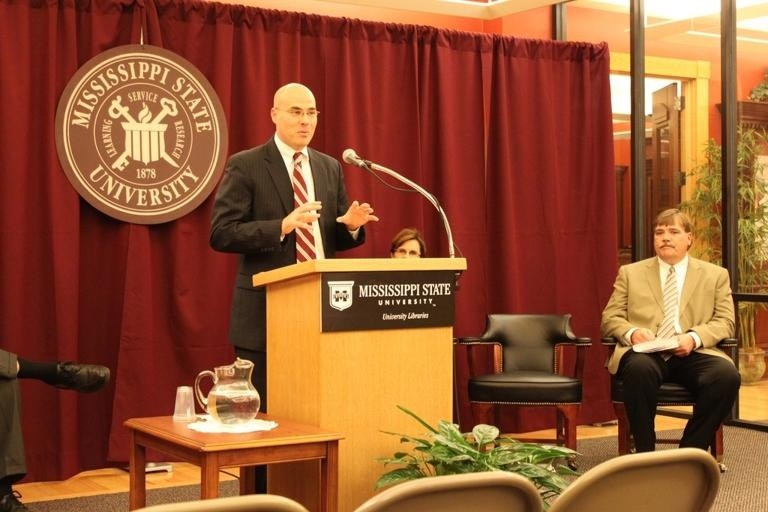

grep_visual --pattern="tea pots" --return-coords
[194,357,261,431]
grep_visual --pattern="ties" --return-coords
[291,151,318,265]
[655,267,679,341]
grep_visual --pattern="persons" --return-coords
[386,226,426,259]
[1,344,115,512]
[206,81,381,497]
[596,206,743,452]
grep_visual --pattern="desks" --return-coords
[124,416,346,512]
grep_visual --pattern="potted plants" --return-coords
[676,125,766,386]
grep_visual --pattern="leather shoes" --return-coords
[50,358,111,395]
[0,488,33,512]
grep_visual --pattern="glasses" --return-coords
[276,107,320,118]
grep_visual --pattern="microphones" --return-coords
[342,148,364,168]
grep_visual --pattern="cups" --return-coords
[174,386,195,423]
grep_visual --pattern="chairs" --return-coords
[130,493,309,512]
[546,447,722,512]
[353,471,544,512]
[603,335,739,474]
[460,312,593,471]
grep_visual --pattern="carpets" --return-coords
[21,419,767,511]
